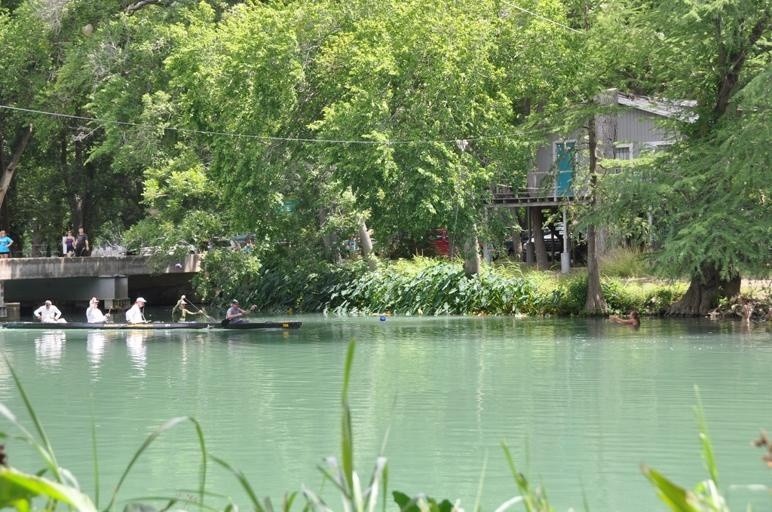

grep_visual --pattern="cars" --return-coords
[123,231,363,257]
[428,222,583,260]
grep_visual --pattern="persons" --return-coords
[171,295,206,322]
[226,299,258,322]
[76,227,89,256]
[86,297,112,323]
[34,300,69,322]
[0,229,15,257]
[64,231,76,257]
[0,232,14,257]
[609,309,641,327]
[126,297,149,323]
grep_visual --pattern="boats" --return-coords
[0,309,304,330]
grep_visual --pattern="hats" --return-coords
[136,298,147,302]
[89,299,99,303]
[178,300,187,304]
[233,301,240,306]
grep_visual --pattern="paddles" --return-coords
[183,299,216,324]
[221,312,250,326]
